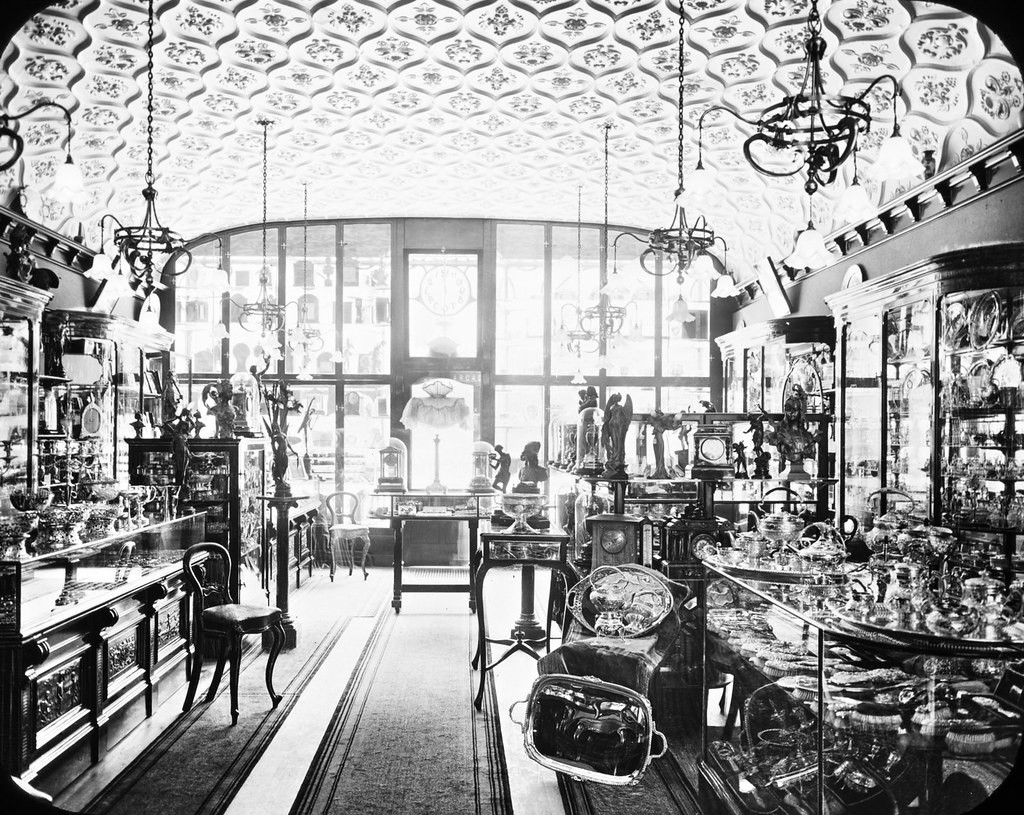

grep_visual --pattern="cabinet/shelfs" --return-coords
[695,560,1024,815]
[125,436,264,658]
[0,508,206,792]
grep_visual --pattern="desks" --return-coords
[470,518,569,712]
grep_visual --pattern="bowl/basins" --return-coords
[717,547,748,565]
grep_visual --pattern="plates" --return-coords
[841,264,862,291]
[970,290,1001,350]
[949,354,1024,409]
[942,302,966,351]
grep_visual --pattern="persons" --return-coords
[781,397,802,430]
[732,442,746,473]
[743,403,768,457]
[490,445,511,492]
[577,386,599,421]
[162,370,181,401]
[163,414,194,484]
[651,409,671,480]
[211,383,235,425]
[270,423,297,483]
[607,394,623,448]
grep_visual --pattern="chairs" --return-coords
[327,493,371,580]
[183,541,283,732]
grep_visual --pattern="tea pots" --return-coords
[943,426,1024,533]
[858,488,915,554]
[850,556,1024,644]
[724,527,767,559]
[748,486,806,545]
[786,536,851,567]
[590,566,664,637]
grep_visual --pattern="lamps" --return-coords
[563,0,924,394]
[84,0,344,390]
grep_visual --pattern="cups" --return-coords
[998,386,1017,410]
